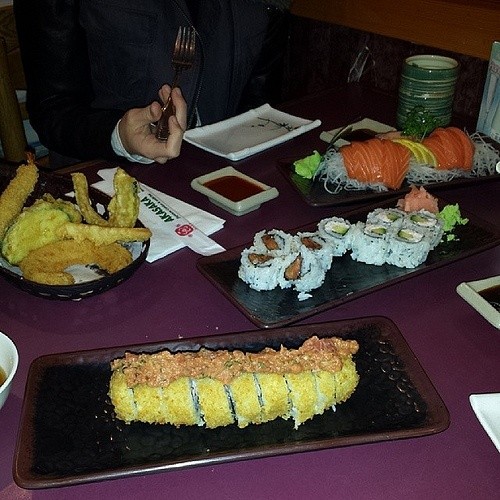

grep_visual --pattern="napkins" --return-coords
[64,179,226,263]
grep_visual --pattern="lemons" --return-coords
[390,139,438,167]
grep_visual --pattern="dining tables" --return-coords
[0,85,499,500]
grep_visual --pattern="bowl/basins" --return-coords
[0,331,19,409]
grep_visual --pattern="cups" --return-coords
[396,55,460,131]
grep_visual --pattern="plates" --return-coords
[191,165,278,216]
[276,127,500,207]
[456,275,500,331]
[197,193,500,328]
[320,118,398,148]
[184,103,322,160]
[0,165,151,299]
[470,393,500,454]
[12,314,451,489]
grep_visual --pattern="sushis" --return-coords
[237,229,332,301]
[107,337,359,430]
[317,207,442,269]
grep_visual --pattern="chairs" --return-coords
[0,5,49,163]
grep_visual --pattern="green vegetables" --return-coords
[396,105,442,142]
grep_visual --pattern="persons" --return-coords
[13,0,289,164]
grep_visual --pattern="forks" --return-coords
[155,26,196,140]
